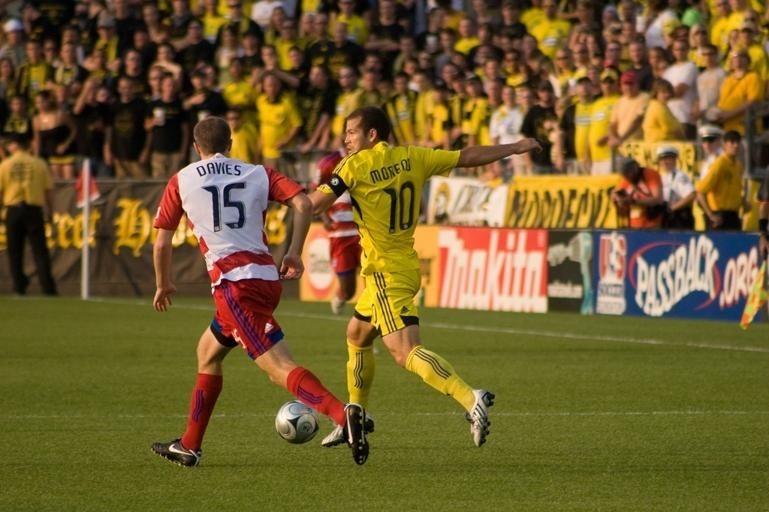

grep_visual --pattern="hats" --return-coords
[656,145,679,160]
[698,125,721,140]
[570,69,639,87]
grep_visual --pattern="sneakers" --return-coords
[343,404,369,465]
[465,389,495,448]
[151,438,202,467]
[321,417,374,448]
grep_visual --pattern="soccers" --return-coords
[275,401,320,443]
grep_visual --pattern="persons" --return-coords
[150,117,373,471]
[0,133,61,296]
[311,134,362,315]
[308,105,542,449]
[0,0,769,258]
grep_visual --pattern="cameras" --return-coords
[645,201,667,220]
